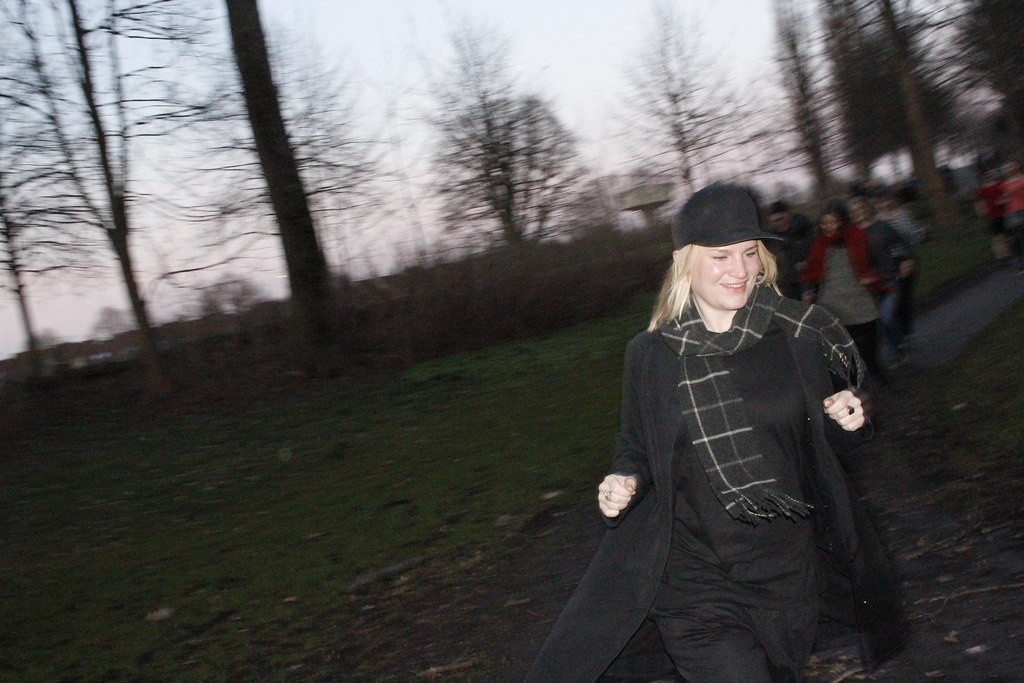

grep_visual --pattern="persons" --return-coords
[764,196,926,395]
[524,183,909,683]
[975,160,1024,267]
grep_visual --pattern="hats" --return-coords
[671,184,784,250]
[765,202,790,221]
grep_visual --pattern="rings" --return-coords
[603,490,611,502]
[847,406,854,415]
